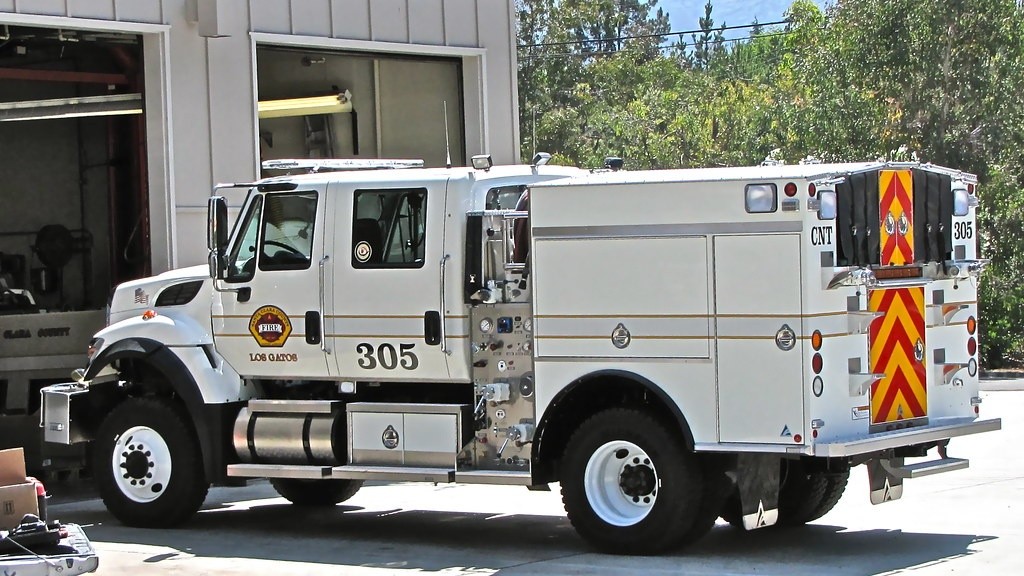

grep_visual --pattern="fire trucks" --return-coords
[39,98,999,559]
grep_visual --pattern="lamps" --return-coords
[257,89,353,120]
[0,92,144,121]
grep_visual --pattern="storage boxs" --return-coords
[0,448,39,532]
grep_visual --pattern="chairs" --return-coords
[356,218,384,264]
